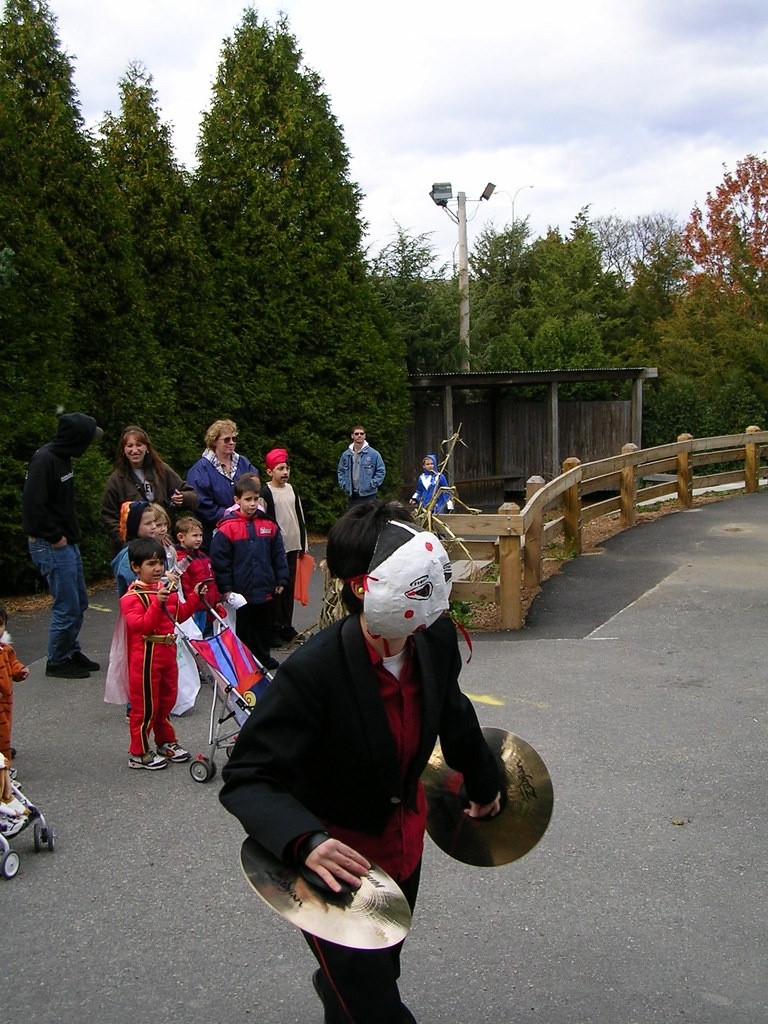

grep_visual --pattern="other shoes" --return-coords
[198,670,214,684]
[289,630,305,641]
[263,657,279,668]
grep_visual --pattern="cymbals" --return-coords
[419,725,557,869]
[238,836,413,951]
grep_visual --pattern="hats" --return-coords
[94,427,103,441]
[265,449,291,469]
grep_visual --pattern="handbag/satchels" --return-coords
[294,553,314,606]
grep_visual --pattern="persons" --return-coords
[0,608,29,793]
[218,501,502,1024]
[409,455,454,514]
[338,426,386,508]
[119,538,192,769]
[99,425,199,557]
[174,516,215,633]
[150,502,183,597]
[265,449,308,649]
[209,473,290,669]
[111,500,156,600]
[186,419,258,554]
[23,413,103,678]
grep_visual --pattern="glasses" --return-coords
[218,436,237,444]
[353,432,365,435]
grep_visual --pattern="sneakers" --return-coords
[72,651,100,671]
[128,751,168,770]
[47,659,90,678]
[157,741,191,762]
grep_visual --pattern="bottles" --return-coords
[167,555,193,579]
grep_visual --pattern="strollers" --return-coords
[161,577,274,782]
[0,782,55,880]
